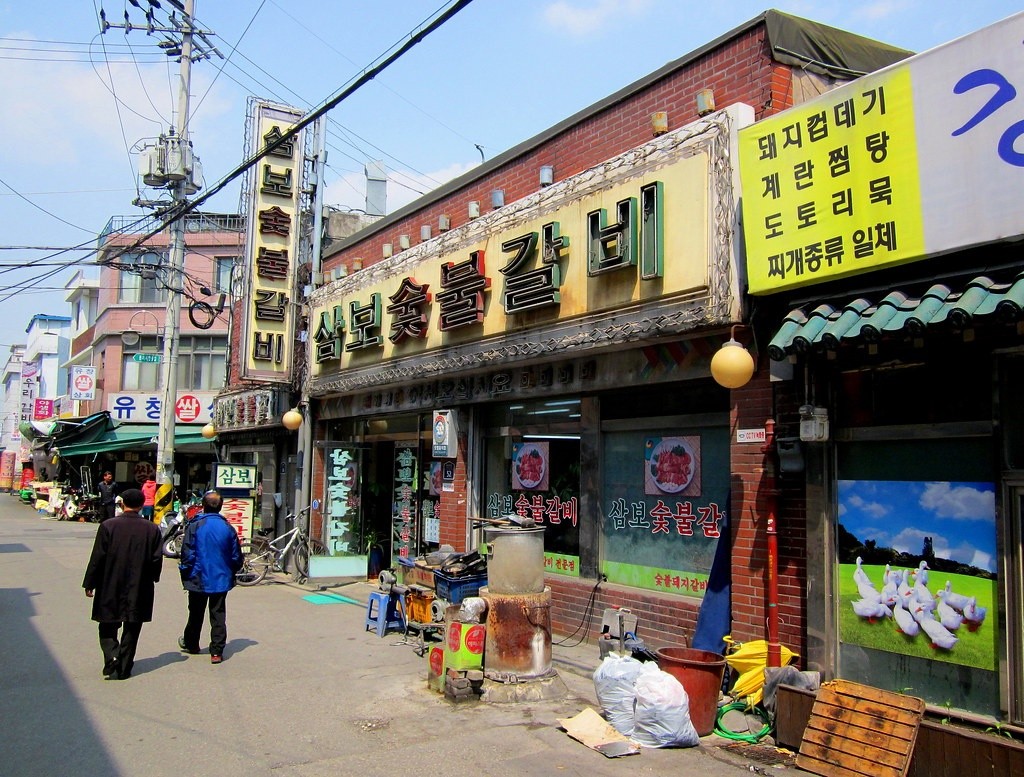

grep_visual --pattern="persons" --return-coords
[83,489,163,680]
[141,471,157,522]
[39,467,48,482]
[178,492,243,664]
[97,471,119,523]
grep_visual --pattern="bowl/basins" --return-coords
[441,548,487,578]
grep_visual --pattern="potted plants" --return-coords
[360,520,390,577]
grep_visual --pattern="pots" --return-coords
[484,526,546,594]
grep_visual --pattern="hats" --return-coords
[123,489,145,508]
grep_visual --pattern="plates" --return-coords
[515,444,546,488]
[431,462,441,495]
[648,438,696,493]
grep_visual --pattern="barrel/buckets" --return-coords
[655,646,727,737]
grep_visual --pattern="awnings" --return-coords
[18,410,219,476]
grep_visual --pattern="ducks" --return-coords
[849,556,986,649]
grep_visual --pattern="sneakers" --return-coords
[177,636,200,654]
[209,642,222,663]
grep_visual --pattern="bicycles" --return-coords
[235,506,330,586]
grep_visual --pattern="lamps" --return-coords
[281,401,310,431]
[201,420,216,439]
[798,405,829,441]
[711,307,759,390]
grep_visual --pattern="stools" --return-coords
[365,592,411,639]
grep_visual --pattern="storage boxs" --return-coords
[427,642,446,694]
[444,604,487,669]
[402,559,488,624]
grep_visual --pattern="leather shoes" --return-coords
[118,672,130,680]
[103,657,119,675]
